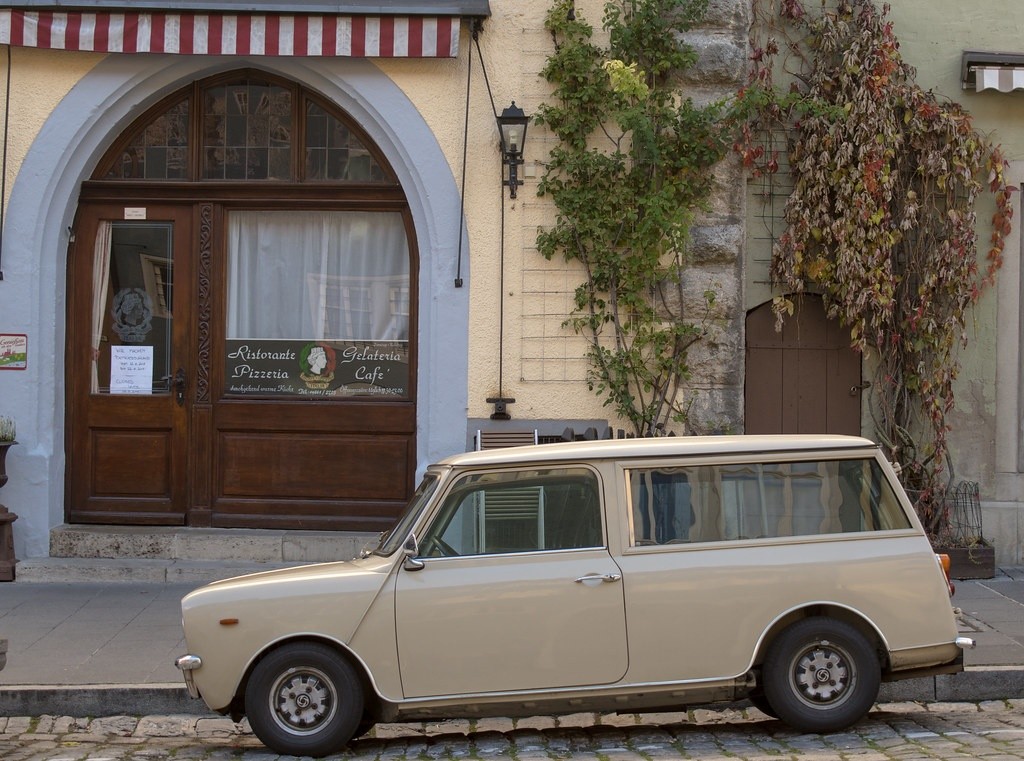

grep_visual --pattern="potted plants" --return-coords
[932,534,996,580]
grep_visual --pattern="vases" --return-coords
[0,440,22,581]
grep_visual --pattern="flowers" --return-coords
[0,414,16,442]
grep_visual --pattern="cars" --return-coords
[176,435,975,757]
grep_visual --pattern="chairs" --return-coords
[545,482,602,549]
[474,430,545,553]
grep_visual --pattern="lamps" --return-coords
[496,100,530,200]
